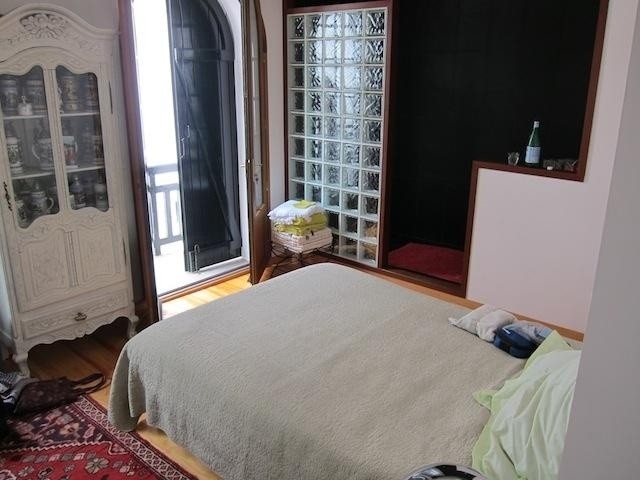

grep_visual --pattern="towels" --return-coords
[265,199,334,255]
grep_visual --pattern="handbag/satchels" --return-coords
[11,372,107,416]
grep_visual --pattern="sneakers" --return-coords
[0,369,25,394]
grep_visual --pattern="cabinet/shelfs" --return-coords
[1,1,142,378]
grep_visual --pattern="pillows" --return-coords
[468,328,582,479]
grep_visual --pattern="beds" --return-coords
[123,261,585,480]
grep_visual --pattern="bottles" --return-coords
[0,69,110,228]
[524,120,543,169]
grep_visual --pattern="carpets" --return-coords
[1,375,204,480]
